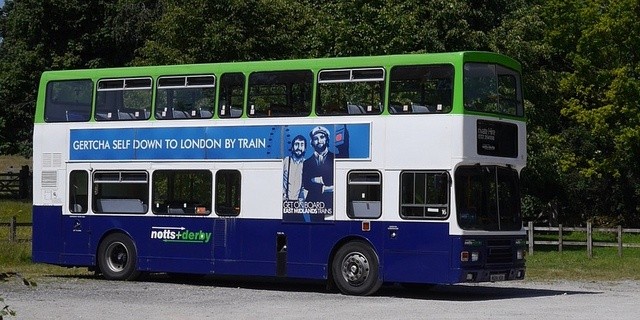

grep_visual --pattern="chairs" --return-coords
[145,108,168,120]
[66,109,90,121]
[198,107,216,119]
[168,203,187,215]
[172,107,193,120]
[118,108,144,120]
[229,106,243,117]
[379,101,404,113]
[194,204,210,216]
[96,108,118,121]
[409,101,431,113]
[348,101,374,114]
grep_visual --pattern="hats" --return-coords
[309,126,331,139]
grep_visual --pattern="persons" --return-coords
[282,134,308,221]
[302,126,334,221]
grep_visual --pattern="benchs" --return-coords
[350,199,381,218]
[94,198,146,214]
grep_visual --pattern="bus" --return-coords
[31,51,528,297]
